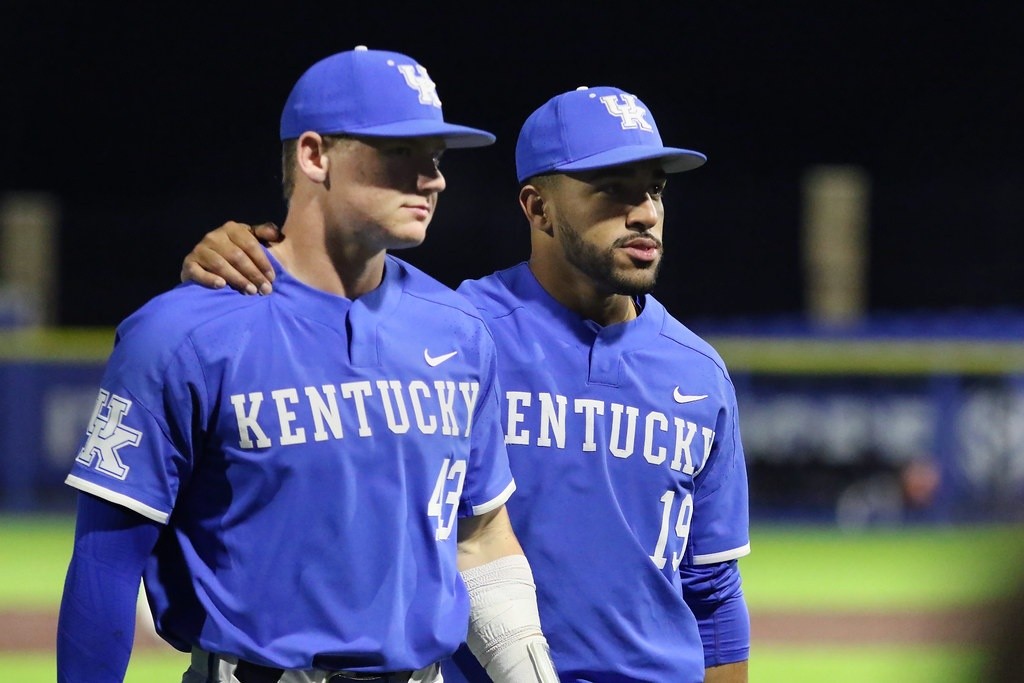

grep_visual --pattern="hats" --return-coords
[279,46,496,149]
[515,86,707,182]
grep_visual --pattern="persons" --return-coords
[56,45,560,683]
[181,87,751,683]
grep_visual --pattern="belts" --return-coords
[207,653,440,683]
